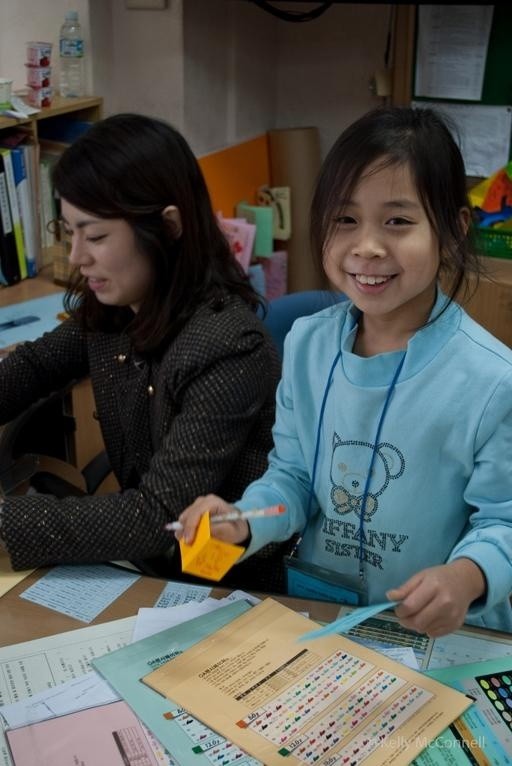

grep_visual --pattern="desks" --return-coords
[0,560,512,766]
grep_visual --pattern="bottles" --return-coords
[58,9,85,98]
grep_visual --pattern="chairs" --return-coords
[80,290,352,498]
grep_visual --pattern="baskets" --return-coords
[471,212,511,258]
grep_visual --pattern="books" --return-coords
[1,117,89,283]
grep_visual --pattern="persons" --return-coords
[1,111,285,574]
[170,102,512,643]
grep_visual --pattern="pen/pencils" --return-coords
[162,504,285,532]
